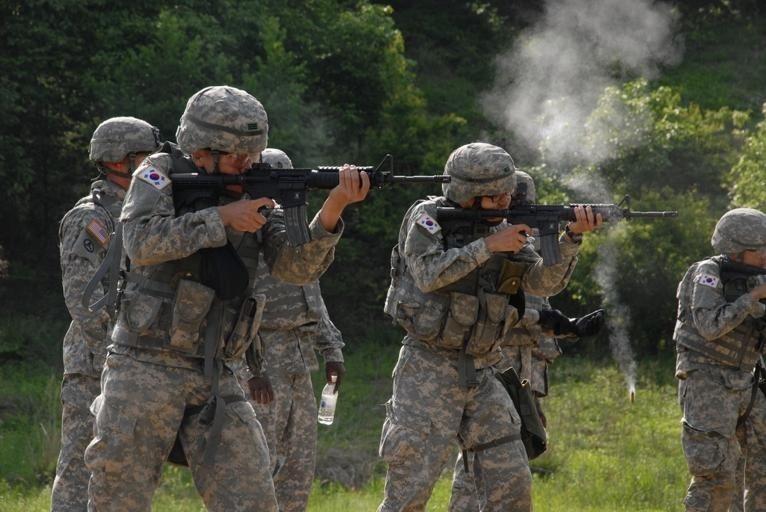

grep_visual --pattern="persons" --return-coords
[51,117,162,511]
[375,141,604,512]
[236,149,347,511]
[86,84,372,512]
[448,171,605,512]
[672,207,766,512]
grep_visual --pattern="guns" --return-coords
[718,257,766,285]
[436,195,679,267]
[168,153,451,246]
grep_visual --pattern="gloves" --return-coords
[535,308,606,338]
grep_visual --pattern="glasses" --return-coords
[482,191,512,203]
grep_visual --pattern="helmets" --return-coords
[511,169,536,204]
[710,207,765,255]
[88,116,161,163]
[441,142,517,204]
[260,146,295,170]
[174,85,270,155]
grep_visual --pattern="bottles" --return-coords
[316,374,339,427]
